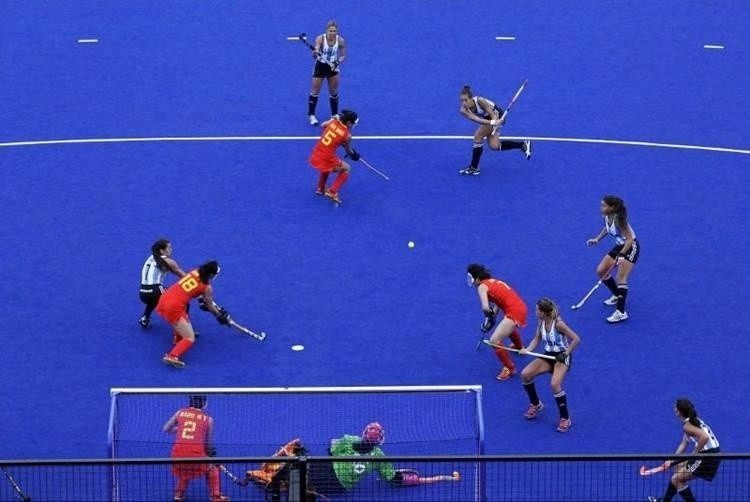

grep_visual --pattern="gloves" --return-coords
[198,297,207,311]
[350,148,359,161]
[481,307,495,332]
[556,352,568,362]
[216,307,230,324]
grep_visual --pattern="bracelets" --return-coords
[490,119,496,126]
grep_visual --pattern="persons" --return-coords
[162,394,231,502]
[648,398,721,501]
[518,296,581,433]
[154,261,232,370]
[307,21,346,126]
[586,195,639,323]
[307,110,360,203]
[246,422,419,494]
[466,263,529,381]
[458,85,531,176]
[137,238,200,337]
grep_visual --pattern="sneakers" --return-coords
[496,367,516,380]
[163,356,184,368]
[525,400,544,418]
[309,114,318,125]
[557,418,571,432]
[316,187,340,203]
[458,166,480,175]
[139,315,150,328]
[604,295,628,323]
[521,139,531,160]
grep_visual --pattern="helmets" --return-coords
[362,422,385,445]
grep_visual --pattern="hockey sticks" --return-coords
[421,471,461,484]
[358,158,390,182]
[483,339,556,360]
[639,460,689,475]
[569,258,619,309]
[213,302,266,342]
[220,464,249,486]
[300,32,338,73]
